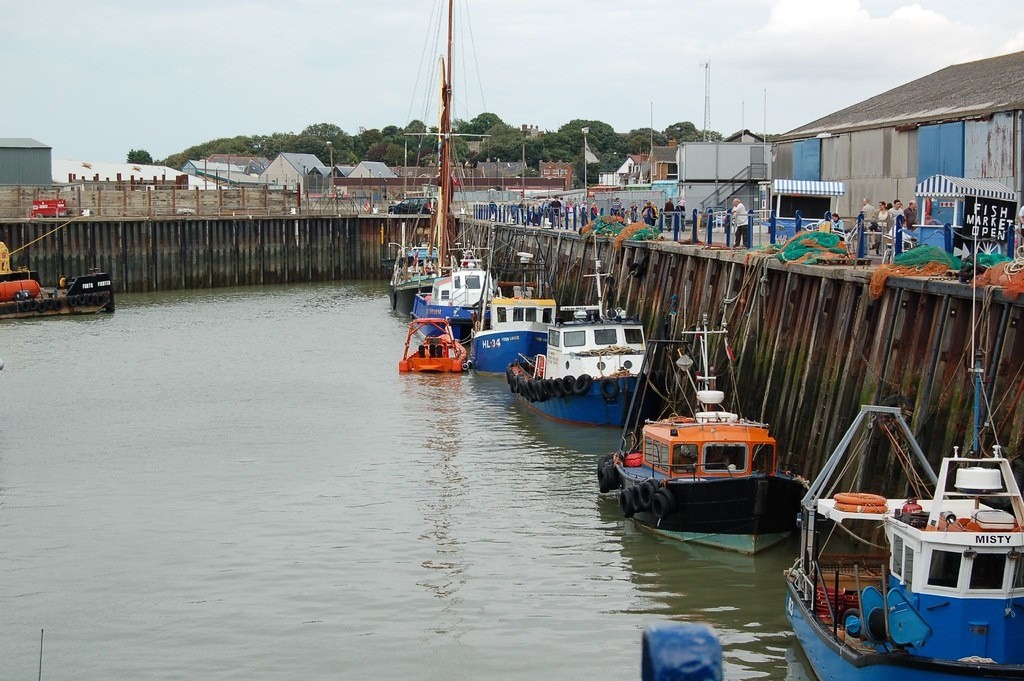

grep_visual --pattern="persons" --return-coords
[548,196,563,229]
[817,214,845,242]
[489,198,497,222]
[663,197,674,231]
[1018,205,1024,253]
[579,200,598,227]
[511,199,539,224]
[565,198,575,228]
[731,198,748,248]
[675,199,686,232]
[559,197,565,227]
[499,202,506,222]
[611,197,659,226]
[855,198,917,256]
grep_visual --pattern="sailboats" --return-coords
[387,1,494,319]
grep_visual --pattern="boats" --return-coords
[407,205,558,377]
[595,263,806,557]
[399,317,474,374]
[784,189,1024,681]
[505,231,654,429]
[0,242,116,320]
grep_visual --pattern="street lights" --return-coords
[581,126,589,201]
[326,141,335,195]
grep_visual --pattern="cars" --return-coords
[487,186,502,192]
[698,206,726,227]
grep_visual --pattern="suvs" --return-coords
[388,197,437,214]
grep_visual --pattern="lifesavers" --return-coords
[38,303,46,312]
[562,376,575,396]
[600,378,618,399]
[552,378,563,397]
[506,363,550,403]
[833,493,887,506]
[546,378,555,397]
[834,502,888,514]
[86,296,94,306]
[20,302,29,311]
[597,453,617,493]
[78,295,86,305]
[55,302,62,310]
[619,478,677,520]
[29,300,38,311]
[68,295,78,307]
[45,300,54,309]
[96,295,104,305]
[573,374,592,395]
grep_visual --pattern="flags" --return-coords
[451,172,462,193]
